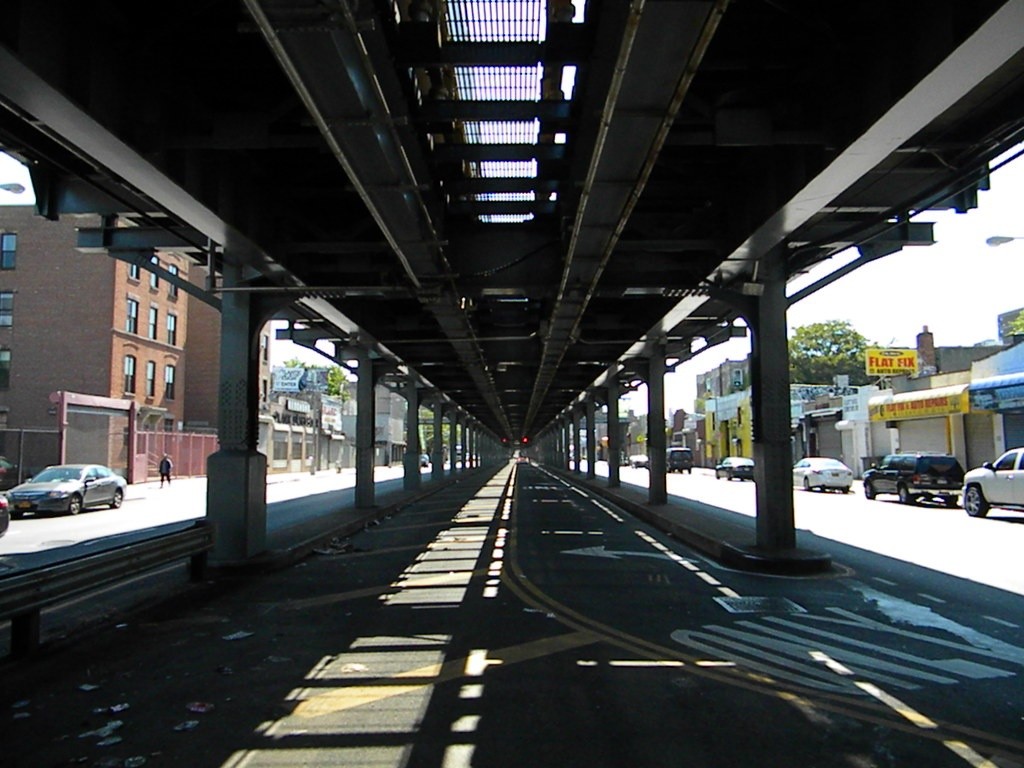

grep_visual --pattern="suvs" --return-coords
[666,447,695,474]
[862,451,965,508]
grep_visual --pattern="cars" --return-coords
[961,447,1024,517]
[0,494,11,539]
[715,457,755,482]
[793,457,853,494]
[6,463,127,520]
[629,455,649,469]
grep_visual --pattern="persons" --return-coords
[159,454,172,489]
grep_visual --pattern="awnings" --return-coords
[969,372,1024,412]
[867,383,968,423]
